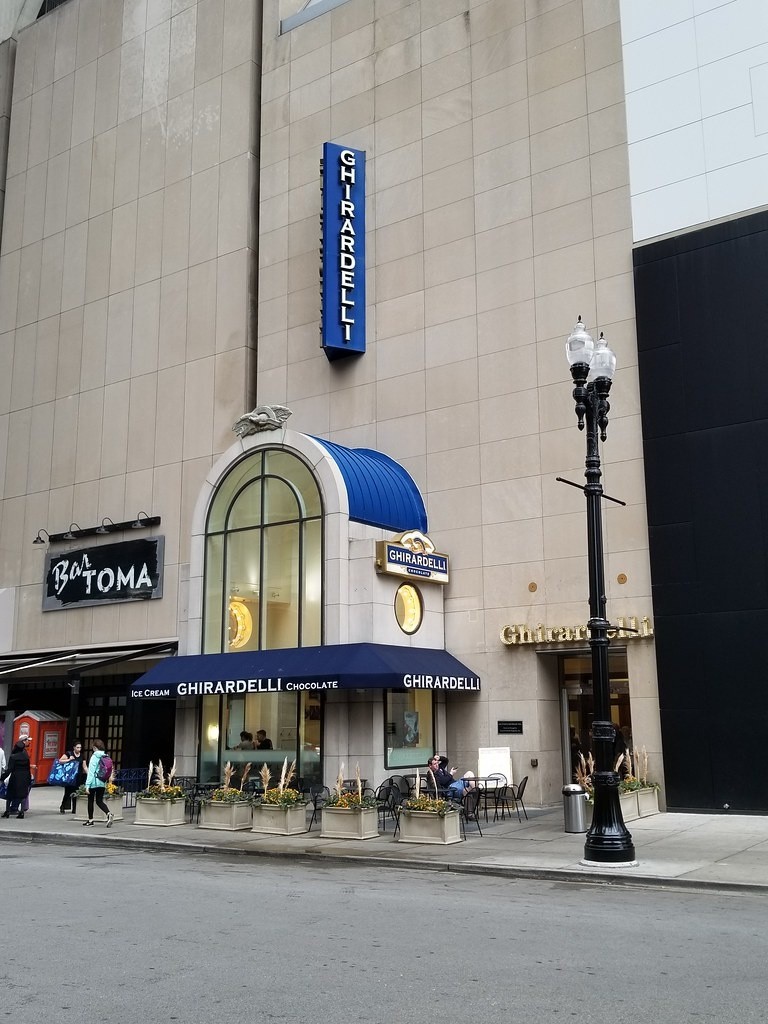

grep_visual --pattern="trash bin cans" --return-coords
[563,783,587,834]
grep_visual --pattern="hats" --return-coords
[19,735,33,741]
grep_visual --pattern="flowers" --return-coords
[253,755,311,813]
[198,759,256,807]
[71,763,128,801]
[321,760,386,812]
[134,757,193,806]
[397,767,465,817]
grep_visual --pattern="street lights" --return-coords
[556,314,639,862]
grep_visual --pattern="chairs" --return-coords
[172,772,529,842]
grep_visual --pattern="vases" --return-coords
[319,807,380,840]
[198,800,253,831]
[397,810,463,845]
[133,798,187,826]
[250,803,307,836]
[73,795,124,822]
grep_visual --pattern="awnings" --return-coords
[130,643,481,699]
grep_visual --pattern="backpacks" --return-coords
[93,753,112,783]
[47,751,79,786]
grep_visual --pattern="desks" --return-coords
[194,782,225,793]
[173,776,198,794]
[336,779,368,795]
[461,777,501,823]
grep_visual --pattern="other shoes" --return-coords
[60,807,65,814]
[82,820,94,827]
[106,813,114,827]
[72,811,76,814]
[468,813,476,821]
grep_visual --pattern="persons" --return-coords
[427,755,478,821]
[59,741,88,813]
[83,740,114,828]
[0,734,37,819]
[613,723,634,779]
[253,730,273,750]
[238,731,254,750]
[570,725,582,754]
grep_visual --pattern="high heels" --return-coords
[1,811,9,818]
[16,812,24,818]
